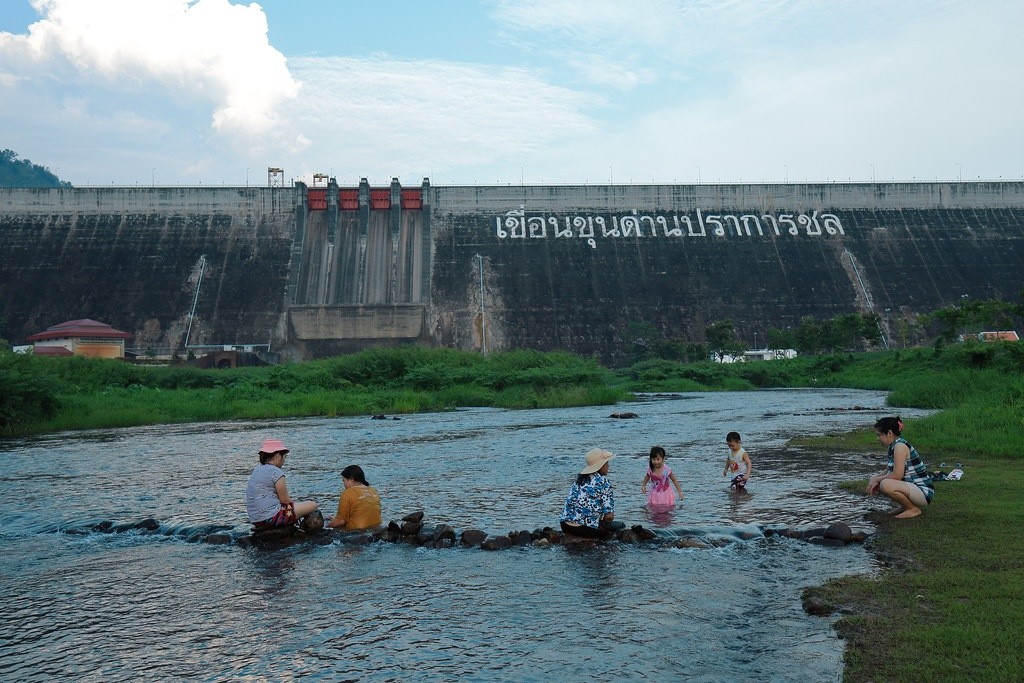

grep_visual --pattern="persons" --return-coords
[323,464,381,532]
[641,446,683,505]
[722,432,752,490]
[865,416,934,519]
[245,438,317,528]
[558,448,626,538]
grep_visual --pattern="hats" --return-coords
[257,439,290,454]
[580,447,616,475]
